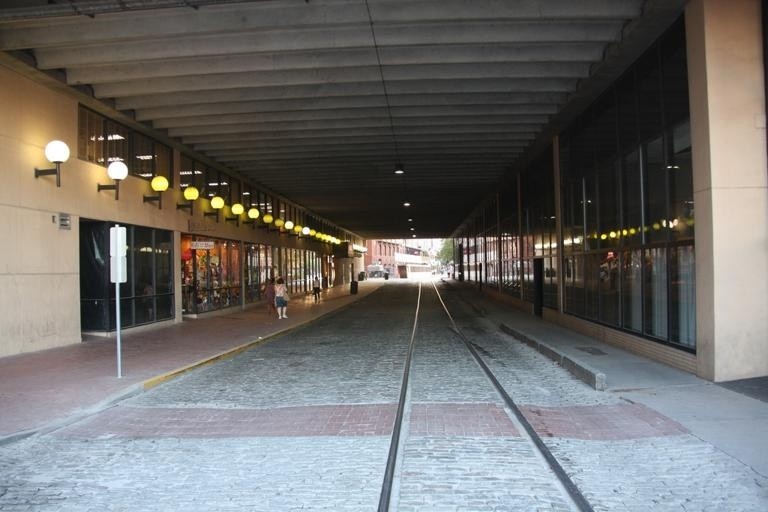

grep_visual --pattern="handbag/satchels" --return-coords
[283,293,290,302]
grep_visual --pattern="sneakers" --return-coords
[278,315,288,319]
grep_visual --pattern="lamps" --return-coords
[175,186,200,217]
[142,176,168,211]
[97,161,128,201]
[33,140,72,188]
[203,196,342,245]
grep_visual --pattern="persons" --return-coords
[274,277,290,319]
[312,276,321,304]
[142,279,159,322]
[264,278,277,317]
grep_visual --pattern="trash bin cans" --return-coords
[350,281,358,294]
[360,272,365,280]
[385,273,388,280]
